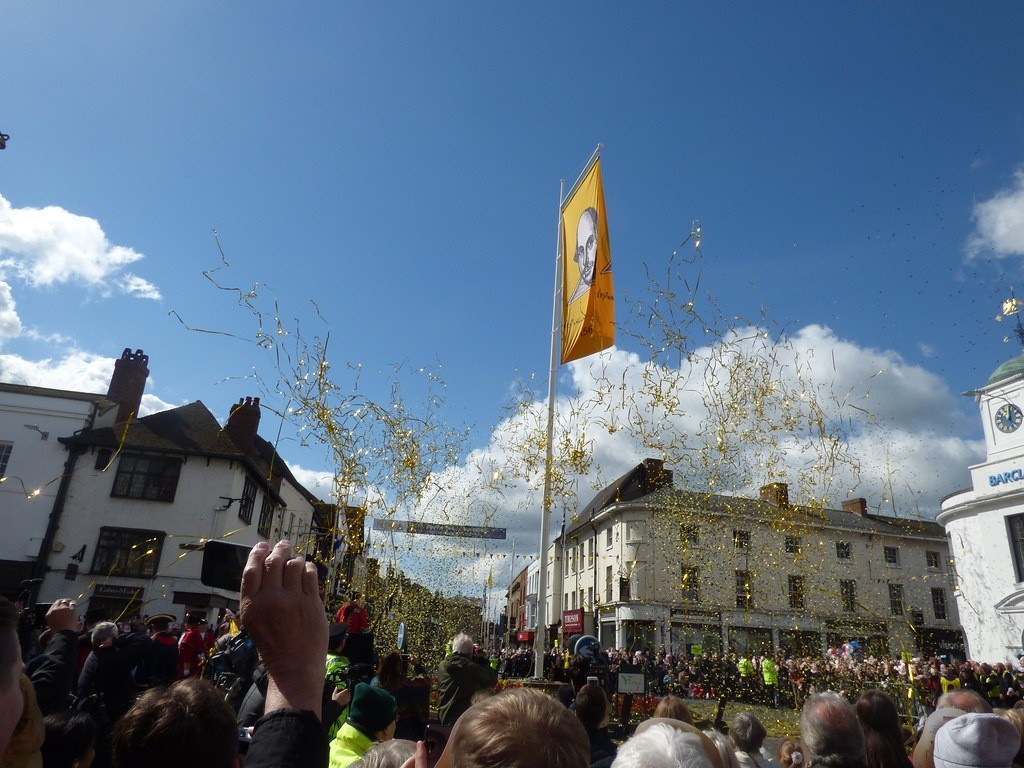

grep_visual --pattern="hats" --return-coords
[350,683,396,736]
[932,713,1020,768]
[144,614,177,625]
[912,707,967,768]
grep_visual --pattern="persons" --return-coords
[0,538,1024,768]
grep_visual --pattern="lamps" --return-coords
[23,423,49,440]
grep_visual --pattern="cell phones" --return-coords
[201,539,254,592]
[424,725,454,768]
[35,603,53,627]
[587,677,598,687]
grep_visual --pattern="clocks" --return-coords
[994,401,1023,433]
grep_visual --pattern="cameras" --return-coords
[400,654,410,661]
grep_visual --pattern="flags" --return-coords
[487,569,493,590]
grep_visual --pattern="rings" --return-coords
[61,601,69,605]
[70,602,77,606]
